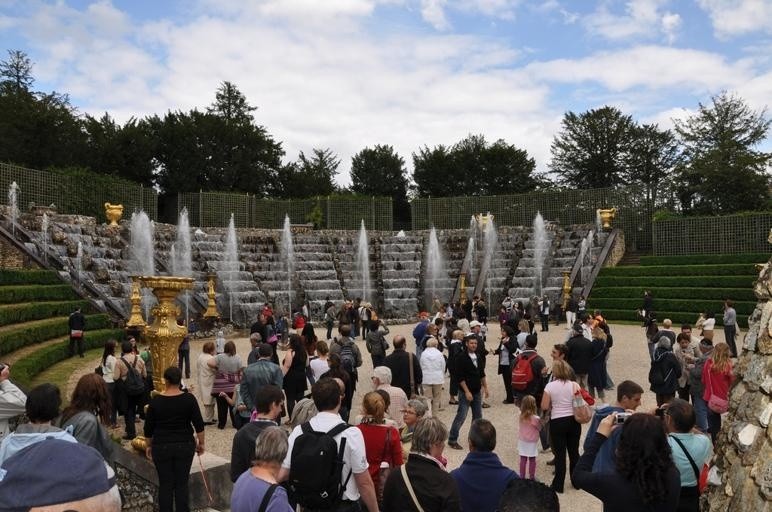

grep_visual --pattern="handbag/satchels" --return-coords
[410,393,429,410]
[572,381,595,424]
[708,394,730,414]
[575,387,594,405]
[71,329,83,338]
[375,426,394,512]
[698,463,710,494]
[95,357,104,377]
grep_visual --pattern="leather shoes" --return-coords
[547,458,555,465]
[448,441,462,449]
[203,422,215,426]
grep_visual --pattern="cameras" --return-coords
[0,363,9,372]
[614,412,633,424]
[656,409,666,416]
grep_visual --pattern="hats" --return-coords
[700,338,712,345]
[469,320,483,328]
[0,436,118,512]
[415,312,428,317]
[572,325,583,333]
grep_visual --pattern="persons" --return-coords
[1,292,740,512]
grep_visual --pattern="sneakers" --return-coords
[449,401,458,404]
[481,402,490,408]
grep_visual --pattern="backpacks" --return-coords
[511,352,538,391]
[649,349,675,386]
[367,307,378,321]
[118,355,145,397]
[336,341,359,383]
[287,421,354,512]
[594,317,612,347]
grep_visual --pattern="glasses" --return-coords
[371,377,375,379]
[403,410,418,415]
